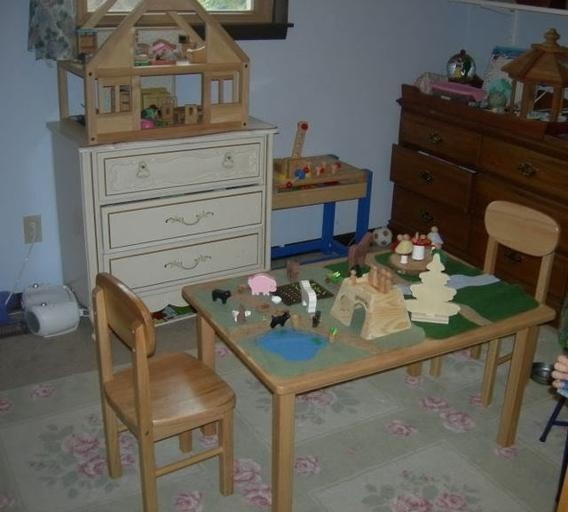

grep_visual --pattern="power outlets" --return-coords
[23,214,43,244]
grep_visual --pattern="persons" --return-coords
[549,354,568,392]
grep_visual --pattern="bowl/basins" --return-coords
[529,362,555,385]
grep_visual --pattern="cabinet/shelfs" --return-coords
[391,83,568,328]
[271,154,374,267]
[47,116,281,342]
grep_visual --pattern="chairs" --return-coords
[427,203,566,407]
[92,272,235,512]
[537,303,568,512]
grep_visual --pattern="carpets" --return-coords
[0,324,566,512]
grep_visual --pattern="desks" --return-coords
[181,247,557,512]
